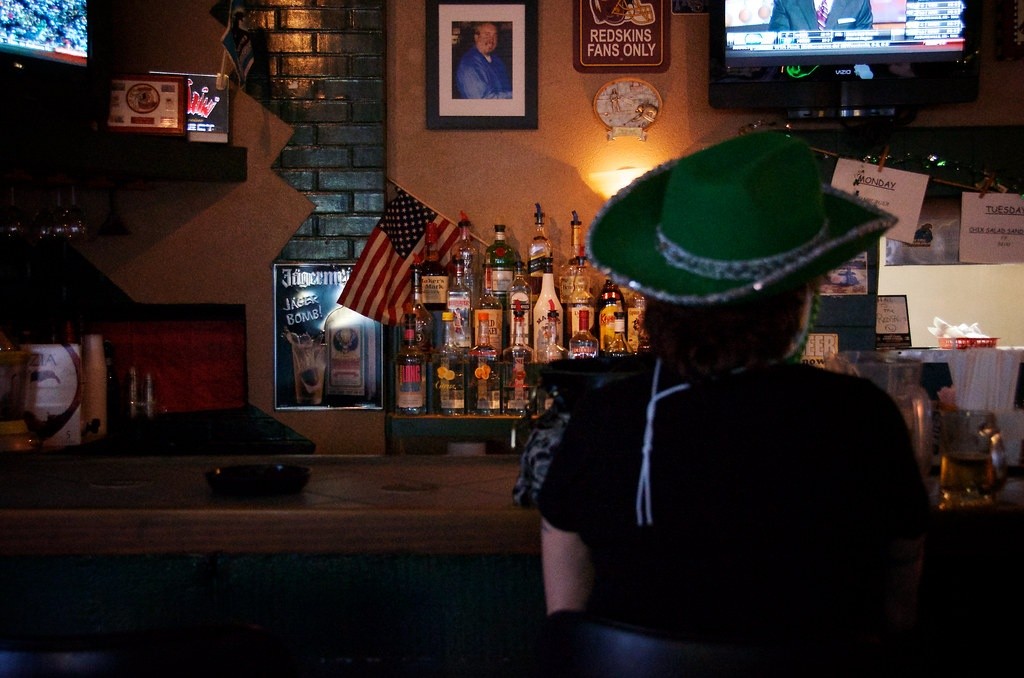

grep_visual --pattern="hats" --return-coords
[585,133,898,307]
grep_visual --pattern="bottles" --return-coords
[396,200,630,416]
[103,340,119,421]
[125,367,155,419]
[323,267,375,402]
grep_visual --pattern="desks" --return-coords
[889,346,1024,411]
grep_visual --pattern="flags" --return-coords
[337,187,461,328]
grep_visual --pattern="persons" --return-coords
[456,23,512,99]
[513,131,931,646]
[768,0,873,32]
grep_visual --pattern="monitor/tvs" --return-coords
[708,0,981,120]
[0,0,95,72]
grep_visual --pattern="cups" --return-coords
[292,343,328,405]
[0,349,32,421]
[81,334,107,440]
[938,410,993,500]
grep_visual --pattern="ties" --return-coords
[817,8,828,26]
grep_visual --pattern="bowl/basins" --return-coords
[204,463,312,496]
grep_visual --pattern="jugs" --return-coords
[835,351,934,478]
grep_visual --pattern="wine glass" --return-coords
[0,181,87,242]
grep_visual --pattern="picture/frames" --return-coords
[425,0,537,130]
